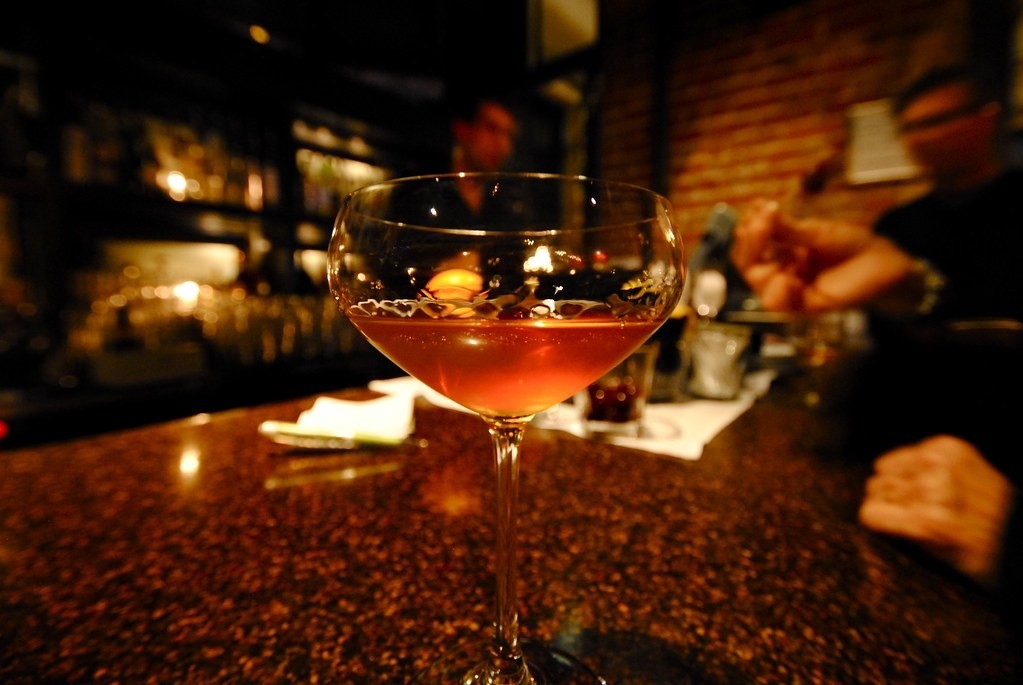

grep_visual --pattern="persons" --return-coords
[728,196,1022,624]
[866,58,1022,322]
[388,100,527,236]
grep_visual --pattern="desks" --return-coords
[0,376,1022,684]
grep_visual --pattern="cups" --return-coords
[578,342,661,437]
[690,320,752,401]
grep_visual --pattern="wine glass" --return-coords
[327,170,685,685]
